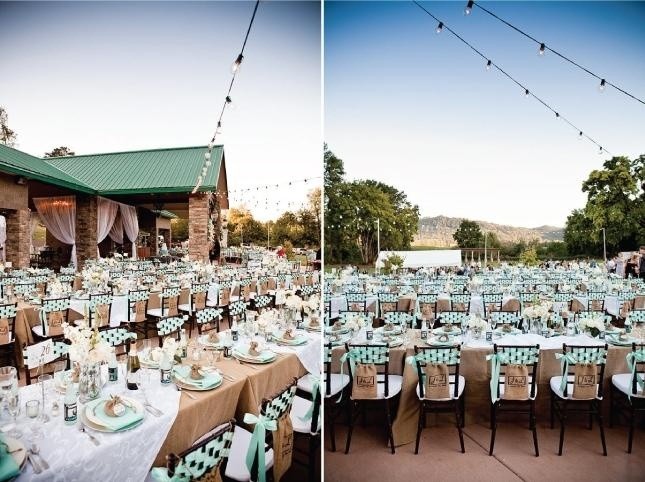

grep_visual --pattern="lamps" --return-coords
[435,22,443,36]
[539,43,545,56]
[463,0,474,16]
[600,79,605,91]
[230,54,243,73]
[486,59,491,71]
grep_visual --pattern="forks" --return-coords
[77,425,100,447]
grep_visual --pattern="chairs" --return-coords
[342,342,403,455]
[610,342,645,454]
[414,345,466,455]
[549,344,608,456]
[489,344,540,457]
[323,343,351,452]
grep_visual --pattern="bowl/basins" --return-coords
[55,383,68,392]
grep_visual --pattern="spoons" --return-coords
[173,384,197,400]
[30,442,49,469]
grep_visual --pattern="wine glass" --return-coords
[490,317,497,339]
[116,363,131,395]
[247,314,254,323]
[26,416,46,442]
[7,395,22,439]
[206,347,218,367]
[0,367,16,409]
[429,317,435,335]
[143,340,153,375]
[460,319,468,341]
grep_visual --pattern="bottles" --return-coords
[107,347,119,383]
[4,286,12,304]
[486,320,492,343]
[231,316,239,341]
[463,283,468,294]
[161,350,171,384]
[421,319,427,341]
[625,314,632,334]
[24,286,30,302]
[180,329,187,357]
[224,332,232,358]
[128,341,140,390]
[366,319,373,343]
[175,331,181,342]
[64,382,77,425]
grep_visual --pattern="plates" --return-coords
[377,326,403,335]
[199,333,233,350]
[274,329,308,345]
[172,365,223,391]
[493,329,525,335]
[324,335,351,346]
[498,328,522,334]
[139,348,177,368]
[233,345,277,364]
[87,397,145,430]
[81,401,144,433]
[31,301,45,306]
[0,436,27,482]
[325,325,350,335]
[368,338,404,348]
[427,334,461,347]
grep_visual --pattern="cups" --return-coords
[54,361,65,392]
[37,374,52,394]
[265,332,273,342]
[27,401,40,418]
[0,402,16,432]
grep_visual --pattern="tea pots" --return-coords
[158,351,172,370]
